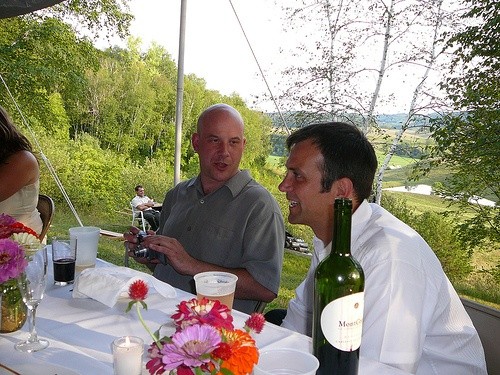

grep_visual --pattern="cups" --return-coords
[253,348,319,375]
[112,336,145,375]
[194,271,238,311]
[69,226,101,272]
[52,239,77,286]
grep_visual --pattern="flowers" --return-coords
[126,280,264,375]
[0,214,40,282]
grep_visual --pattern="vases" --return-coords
[0,278,29,332]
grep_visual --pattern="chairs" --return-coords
[460,297,500,375]
[36,194,54,243]
[130,202,148,232]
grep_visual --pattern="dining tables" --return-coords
[0,259,414,375]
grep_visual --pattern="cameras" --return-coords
[133,230,168,266]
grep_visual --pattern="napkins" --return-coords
[80,266,176,310]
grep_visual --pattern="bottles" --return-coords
[311,196,365,375]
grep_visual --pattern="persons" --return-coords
[132,186,161,232]
[278,122,487,375]
[124,103,285,316]
[0,106,43,237]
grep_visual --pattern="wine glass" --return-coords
[15,243,49,352]
